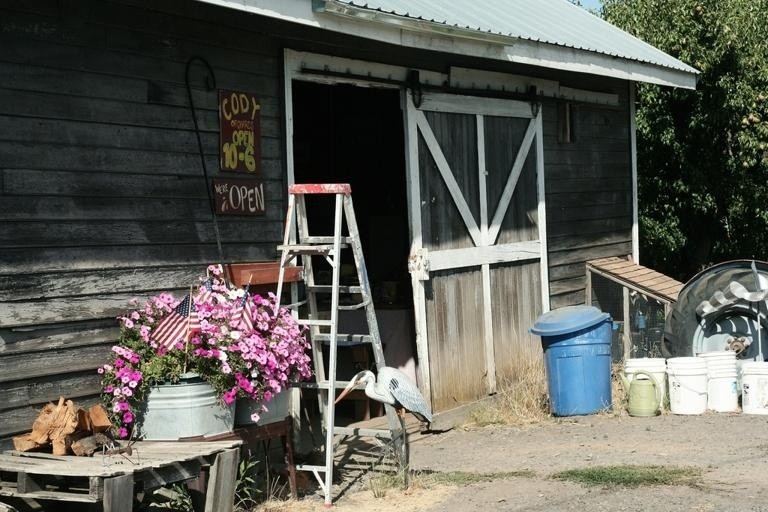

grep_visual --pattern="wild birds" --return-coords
[332,363,436,504]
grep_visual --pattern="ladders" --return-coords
[258,182,409,503]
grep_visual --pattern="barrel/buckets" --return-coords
[624,351,768,415]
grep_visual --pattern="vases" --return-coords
[137,378,237,440]
[238,380,292,428]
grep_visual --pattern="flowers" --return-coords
[76,269,311,440]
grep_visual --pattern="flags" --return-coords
[150,293,202,351]
[229,293,253,332]
[196,278,214,308]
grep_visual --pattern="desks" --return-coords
[209,416,301,507]
